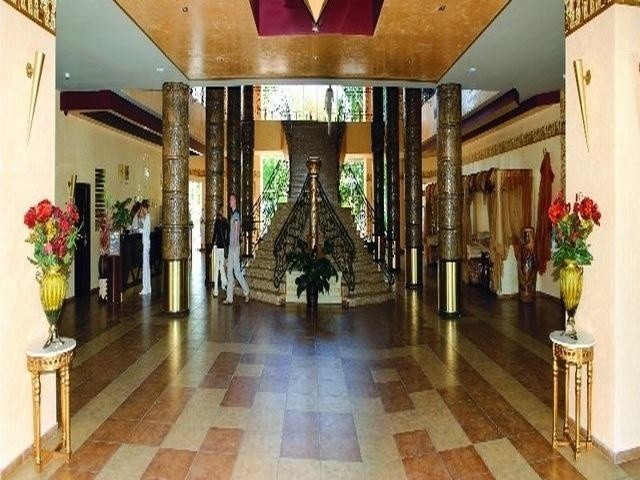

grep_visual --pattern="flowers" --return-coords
[548,190,602,267]
[24,196,88,283]
[95,205,110,256]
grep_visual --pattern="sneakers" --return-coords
[139,290,151,295]
[213,286,250,304]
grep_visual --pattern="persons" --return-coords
[130,201,141,231]
[223,192,251,305]
[208,203,228,297]
[138,199,156,295]
[325,84,335,136]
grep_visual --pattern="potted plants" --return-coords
[286,235,338,309]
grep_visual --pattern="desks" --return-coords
[25,336,77,474]
[548,331,596,461]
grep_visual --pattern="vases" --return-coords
[40,264,69,348]
[560,258,584,341]
[98,254,109,278]
